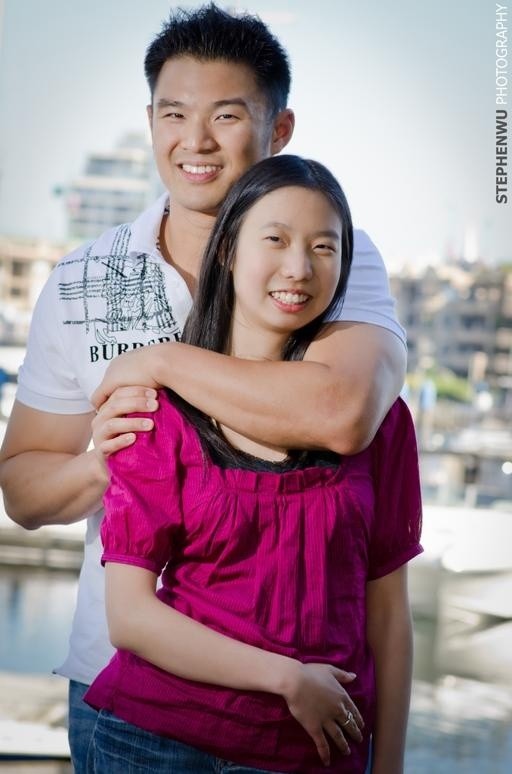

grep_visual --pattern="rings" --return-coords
[341,712,353,726]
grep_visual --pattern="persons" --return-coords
[0,0,408,774]
[83,154,424,774]
